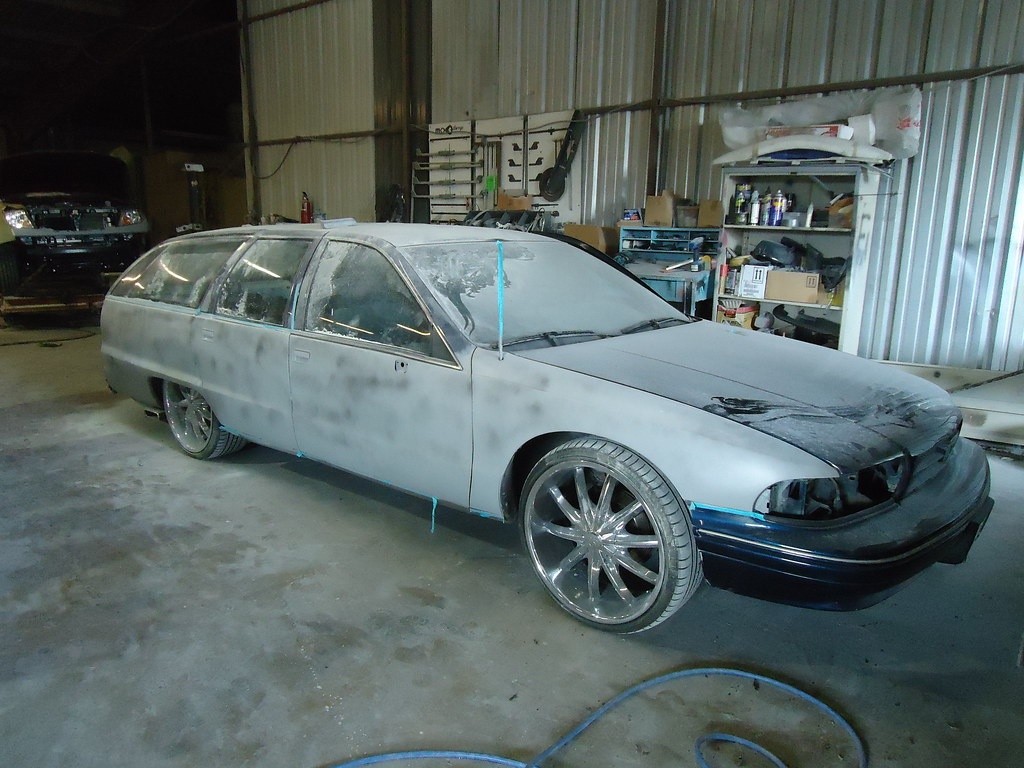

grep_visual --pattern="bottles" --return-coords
[734,182,795,227]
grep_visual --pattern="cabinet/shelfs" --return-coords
[711,165,883,356]
[617,225,720,316]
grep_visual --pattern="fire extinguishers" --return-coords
[300,191,311,224]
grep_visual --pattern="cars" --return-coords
[100,217,996,639]
[0,147,158,322]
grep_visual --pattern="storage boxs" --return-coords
[741,265,775,299]
[617,220,643,227]
[622,208,645,220]
[782,212,806,228]
[829,212,854,230]
[764,268,819,304]
[644,195,691,228]
[563,224,618,257]
[697,199,723,227]
[715,303,761,330]
[676,205,699,228]
[498,187,532,210]
